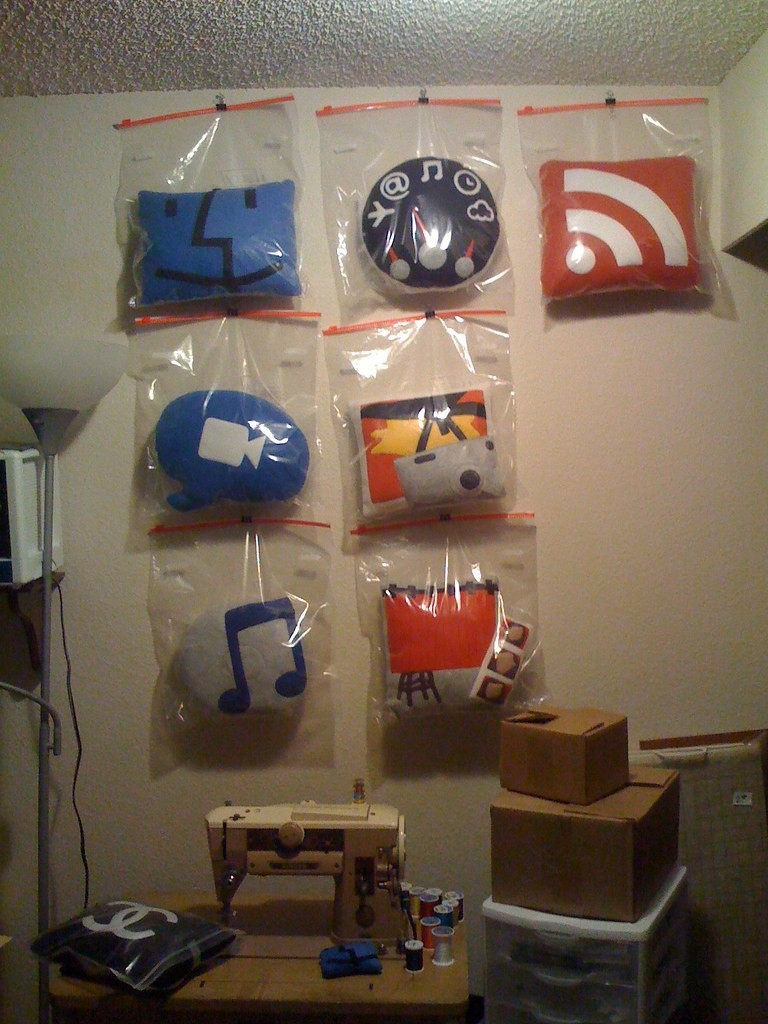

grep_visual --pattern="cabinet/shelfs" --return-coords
[481,864,688,1024]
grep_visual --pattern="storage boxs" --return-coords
[498,705,630,806]
[490,766,682,924]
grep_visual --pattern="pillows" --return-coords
[348,381,507,518]
[179,598,316,715]
[538,154,700,301]
[379,574,502,726]
[132,179,302,309]
[29,897,239,992]
[359,155,501,294]
[153,389,311,512]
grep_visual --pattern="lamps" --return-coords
[0,336,130,1023]
